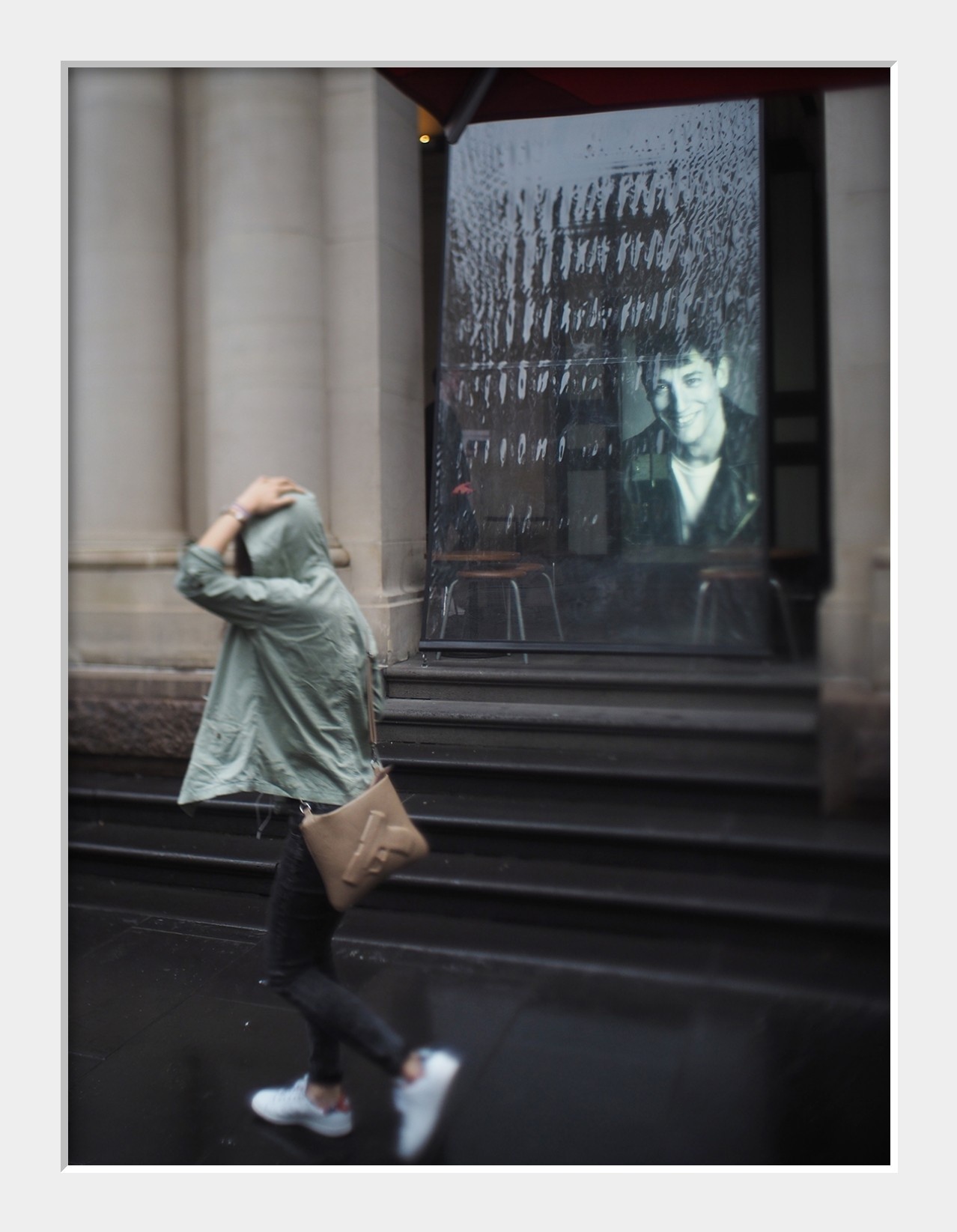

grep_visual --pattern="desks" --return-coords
[430,548,521,659]
[710,544,813,653]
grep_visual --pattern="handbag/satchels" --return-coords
[299,767,429,911]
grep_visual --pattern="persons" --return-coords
[172,475,461,1157]
[617,322,761,546]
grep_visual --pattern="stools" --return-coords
[430,563,564,665]
[688,566,804,665]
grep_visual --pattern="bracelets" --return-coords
[224,503,251,524]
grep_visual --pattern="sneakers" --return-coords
[393,1048,459,1160]
[251,1073,353,1137]
